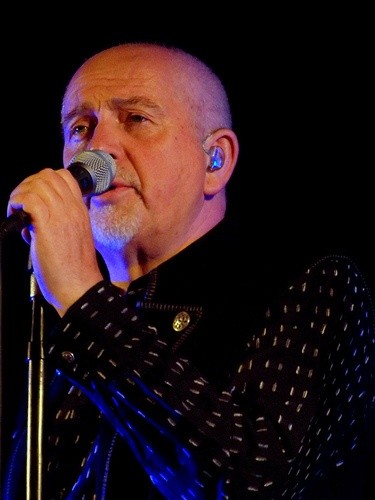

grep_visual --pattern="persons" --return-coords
[2,43,374,500]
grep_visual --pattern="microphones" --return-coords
[0,149,117,234]
